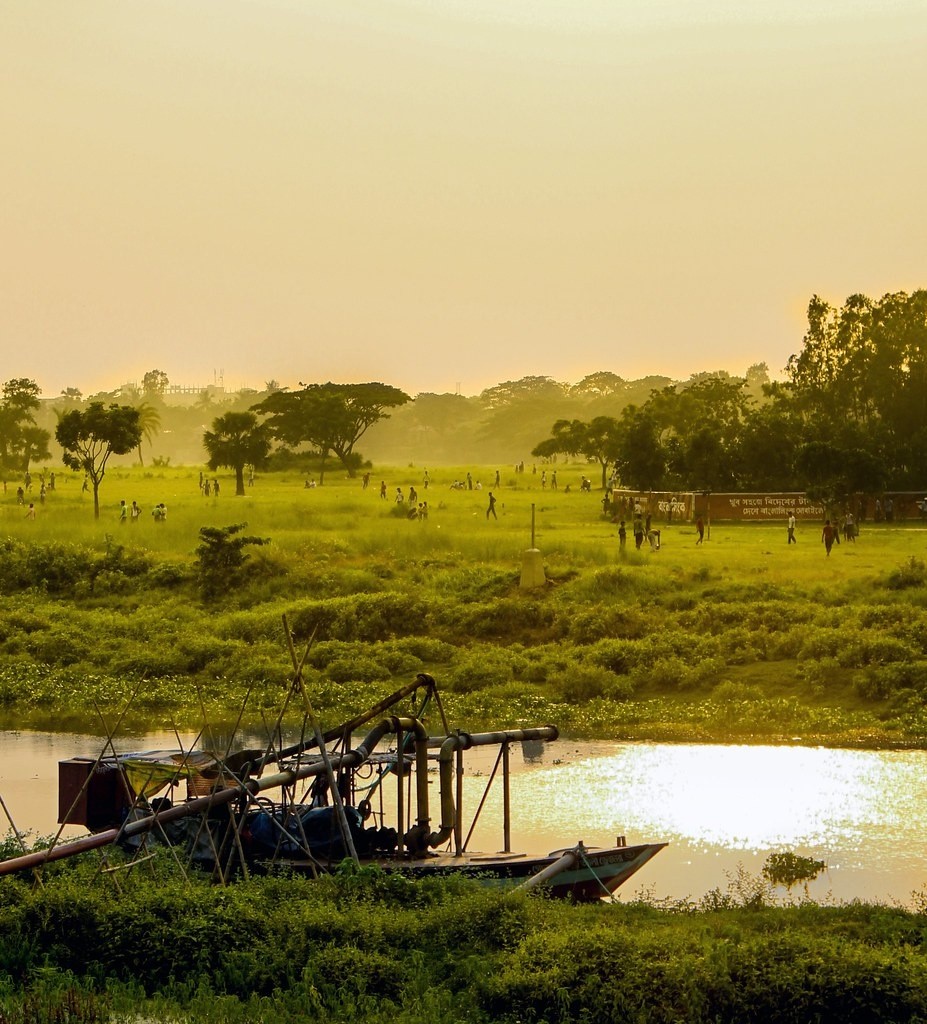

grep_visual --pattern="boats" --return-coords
[55,669,670,901]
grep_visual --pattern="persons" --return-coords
[667,499,674,522]
[634,501,642,517]
[648,528,661,551]
[152,797,173,814]
[821,494,927,557]
[118,499,168,525]
[2,467,89,519]
[644,513,652,541]
[206,785,253,860]
[196,460,635,523]
[695,514,705,545]
[618,521,627,551]
[633,514,646,549]
[787,511,798,544]
